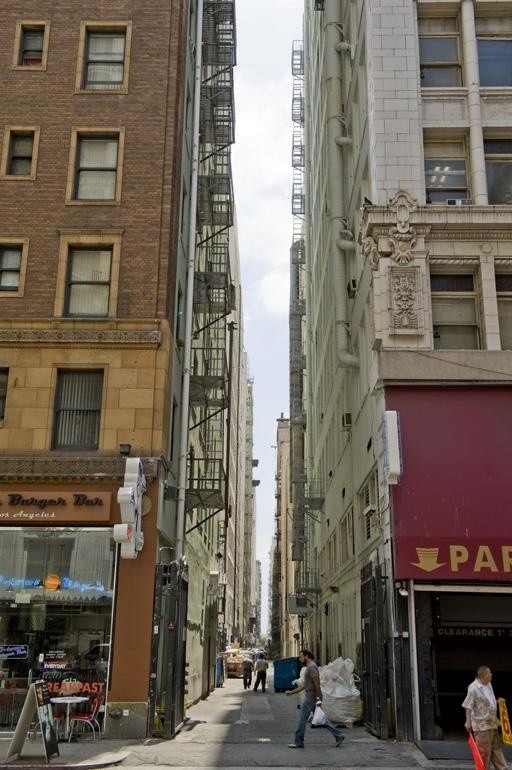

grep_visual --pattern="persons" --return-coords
[239,654,254,690]
[462,665,509,770]
[252,654,269,693]
[284,650,345,749]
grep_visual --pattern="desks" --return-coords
[49,696,90,740]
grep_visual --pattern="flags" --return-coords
[467,735,485,770]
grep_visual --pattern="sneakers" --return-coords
[287,743,304,749]
[335,735,345,747]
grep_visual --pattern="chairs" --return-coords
[0,691,16,728]
[68,696,103,744]
[31,712,64,744]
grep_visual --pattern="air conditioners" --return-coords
[446,199,473,205]
[347,279,357,298]
[342,412,351,427]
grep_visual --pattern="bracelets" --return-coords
[315,697,320,700]
